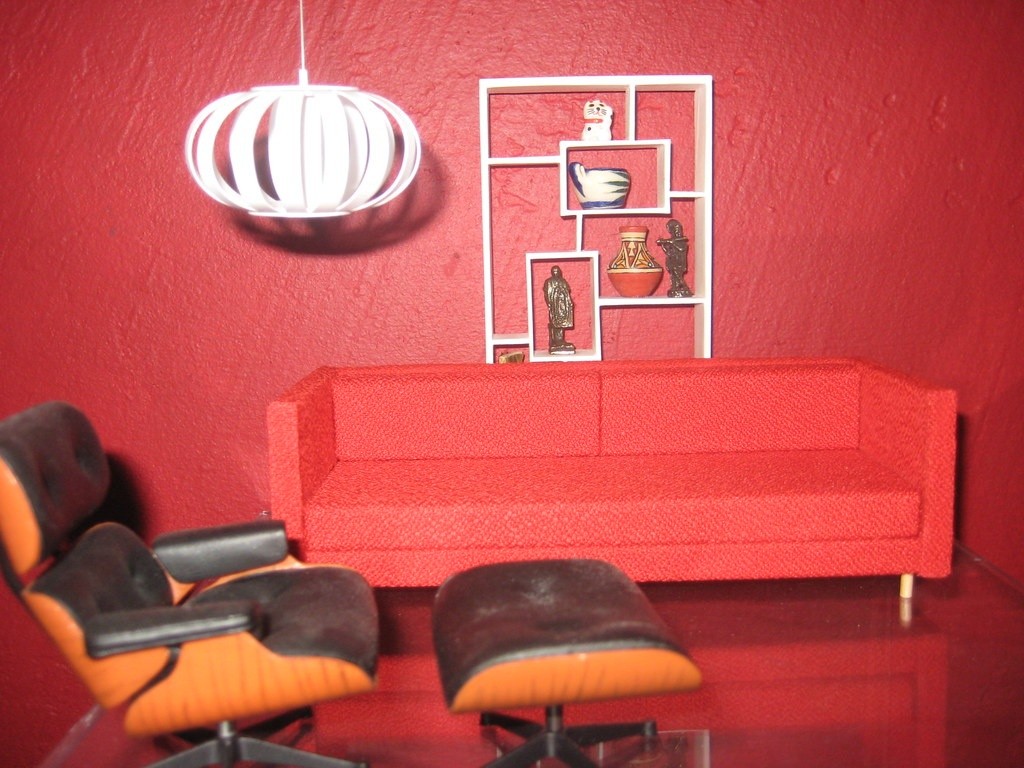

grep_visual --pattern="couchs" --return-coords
[309,588,948,768]
[266,354,958,598]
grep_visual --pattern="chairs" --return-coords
[0,399,382,768]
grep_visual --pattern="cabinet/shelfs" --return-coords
[478,73,713,364]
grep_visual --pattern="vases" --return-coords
[606,226,662,296]
[568,162,631,210]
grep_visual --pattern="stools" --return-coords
[429,555,709,768]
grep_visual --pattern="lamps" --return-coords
[184,1,423,220]
